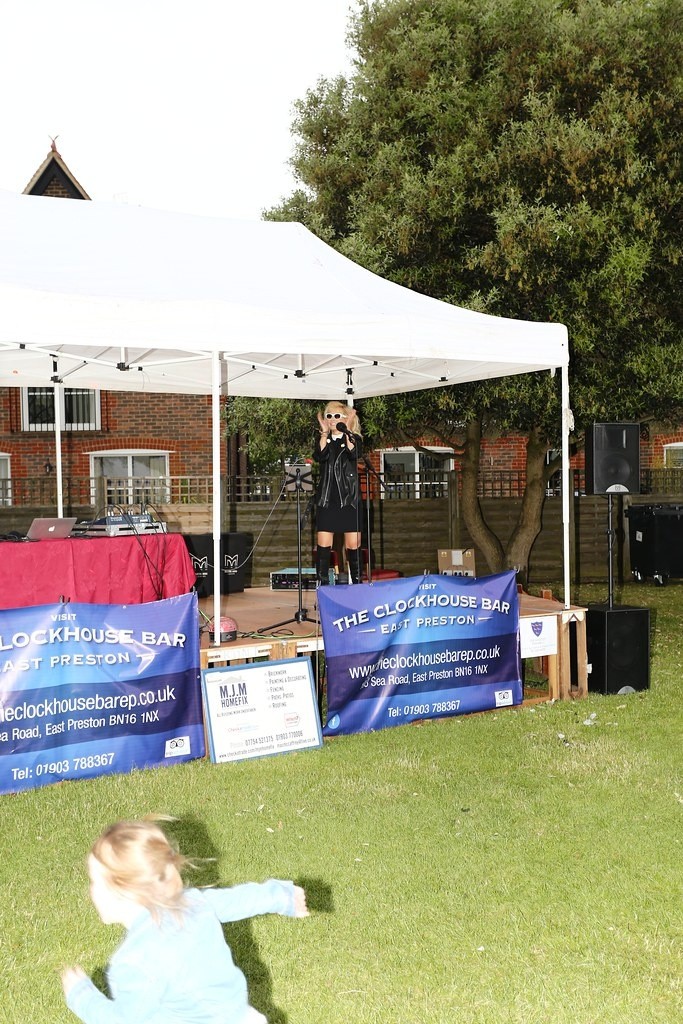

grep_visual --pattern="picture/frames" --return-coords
[200,656,323,764]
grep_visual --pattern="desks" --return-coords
[0,533,196,612]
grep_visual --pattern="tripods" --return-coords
[257,469,322,633]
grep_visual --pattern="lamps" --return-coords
[43,458,53,474]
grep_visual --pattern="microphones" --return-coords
[336,422,354,439]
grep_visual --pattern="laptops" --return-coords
[21,517,78,541]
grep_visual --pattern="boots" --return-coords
[345,548,362,583]
[317,545,332,586]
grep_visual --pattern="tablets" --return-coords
[285,464,313,491]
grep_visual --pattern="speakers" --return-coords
[181,532,214,598]
[569,604,651,696]
[220,532,254,594]
[584,423,640,496]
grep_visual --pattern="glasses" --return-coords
[324,413,348,420]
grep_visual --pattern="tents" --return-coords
[0,190,572,646]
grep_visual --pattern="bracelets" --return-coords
[319,431,329,435]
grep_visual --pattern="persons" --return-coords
[312,401,364,587]
[60,811,313,1024]
